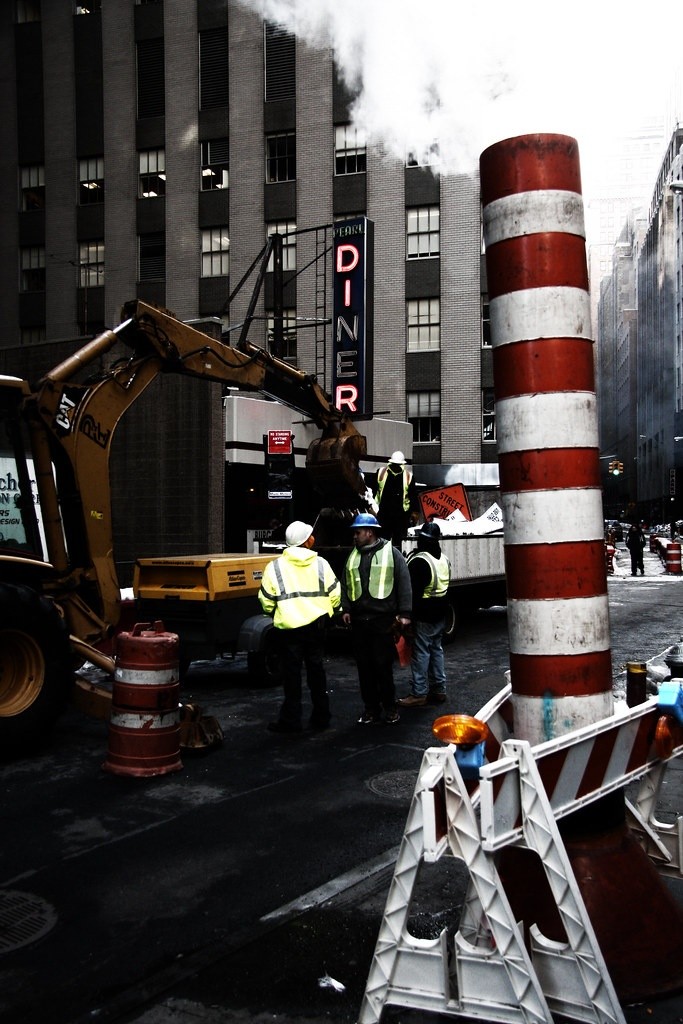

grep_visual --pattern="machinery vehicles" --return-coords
[0,222,368,752]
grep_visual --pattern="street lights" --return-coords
[670,497,675,540]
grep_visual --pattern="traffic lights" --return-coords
[608,459,623,479]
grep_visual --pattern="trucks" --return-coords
[262,460,506,642]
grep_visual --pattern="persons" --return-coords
[340,511,414,728]
[626,524,647,576]
[366,450,421,533]
[254,516,351,733]
[394,520,455,707]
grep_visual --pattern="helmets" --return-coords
[284,520,315,549]
[387,453,407,465]
[418,523,441,542]
[350,514,383,532]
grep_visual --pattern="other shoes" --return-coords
[398,691,429,707]
[631,571,636,575]
[298,722,330,739]
[357,703,380,725]
[641,569,644,574]
[429,688,448,704]
[266,719,302,733]
[385,703,402,724]
